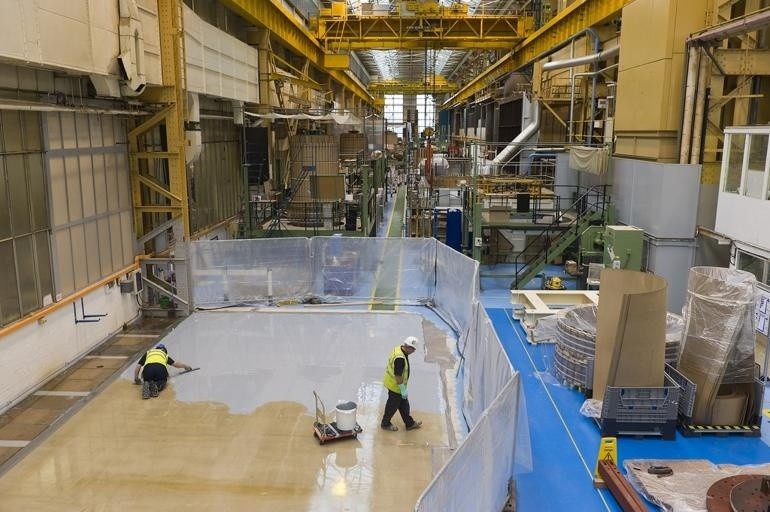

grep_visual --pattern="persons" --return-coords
[381,335,424,431]
[133,343,192,400]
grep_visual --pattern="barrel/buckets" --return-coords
[335,400,358,434]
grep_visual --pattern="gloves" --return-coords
[398,383,408,399]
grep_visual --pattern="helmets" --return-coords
[157,344,167,353]
[405,337,417,350]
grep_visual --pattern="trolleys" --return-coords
[312,389,364,446]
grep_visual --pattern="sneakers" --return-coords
[143,383,150,399]
[150,384,158,397]
[407,421,422,430]
[381,424,398,431]
